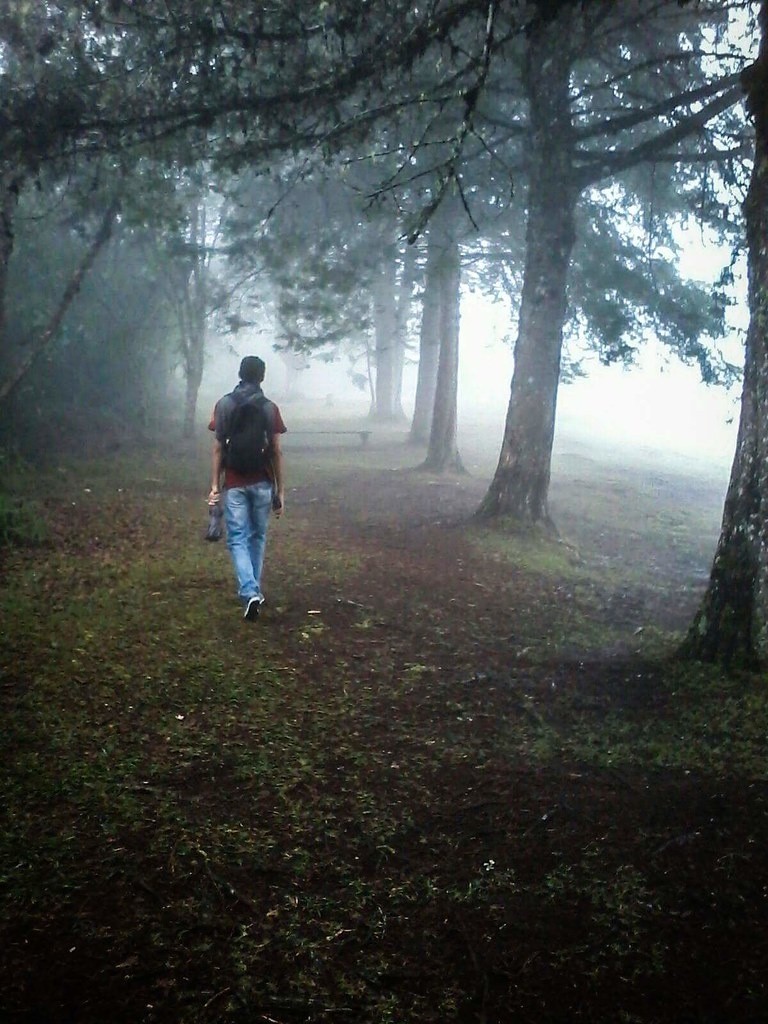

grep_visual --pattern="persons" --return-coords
[205,355,287,623]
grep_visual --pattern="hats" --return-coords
[238,355,266,382]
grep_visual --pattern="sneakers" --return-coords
[244,596,261,621]
[258,592,266,605]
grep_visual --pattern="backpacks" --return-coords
[221,392,270,472]
